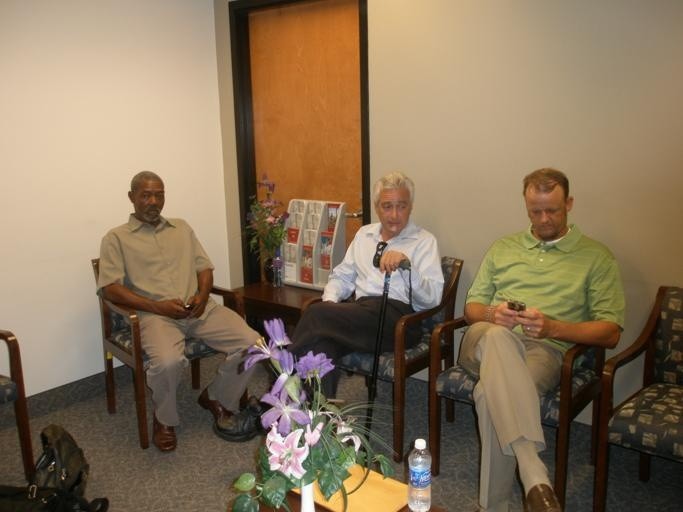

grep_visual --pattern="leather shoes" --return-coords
[152,410,176,451]
[197,387,224,416]
[523,483,562,512]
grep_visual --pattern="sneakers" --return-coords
[214,396,269,443]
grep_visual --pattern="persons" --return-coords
[94,170,263,451]
[457,167,628,512]
[289,170,445,407]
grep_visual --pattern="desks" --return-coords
[254,482,446,512]
[229,279,336,326]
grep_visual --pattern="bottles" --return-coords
[271,251,282,288]
[407,438,435,512]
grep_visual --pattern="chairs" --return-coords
[0,328,34,483]
[422,309,612,512]
[296,253,465,463]
[595,280,683,511]
[89,254,254,451]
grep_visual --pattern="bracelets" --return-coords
[485,307,493,322]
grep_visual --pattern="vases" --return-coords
[297,479,317,512]
[261,258,277,284]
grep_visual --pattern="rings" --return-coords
[527,326,530,331]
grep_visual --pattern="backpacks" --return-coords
[30,423,90,498]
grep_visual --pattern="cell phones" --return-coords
[508,301,525,311]
[184,304,191,308]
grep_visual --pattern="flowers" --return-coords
[223,311,399,512]
[242,172,291,267]
